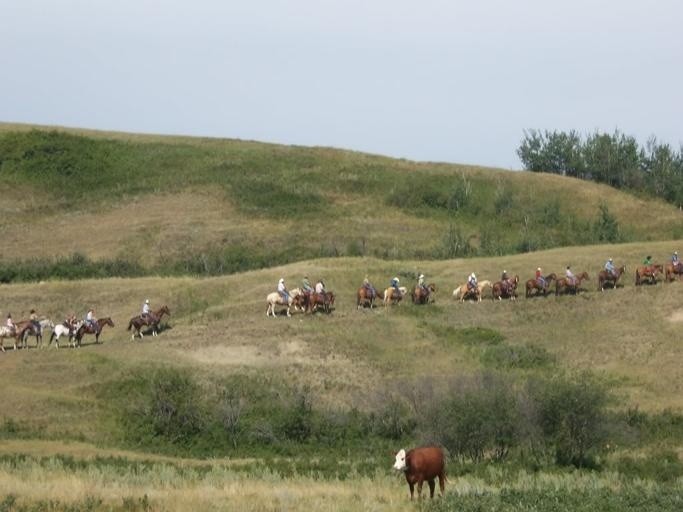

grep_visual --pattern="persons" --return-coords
[535,268,546,289]
[501,269,512,287]
[66,311,78,332]
[278,276,328,306]
[390,276,401,299]
[142,299,156,323]
[362,274,376,300]
[6,313,17,338]
[604,257,615,273]
[468,272,478,290]
[643,255,654,266]
[565,266,576,286]
[670,251,678,267]
[29,308,42,336]
[418,275,431,294]
[85,307,100,334]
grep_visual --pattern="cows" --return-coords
[390,446,451,501]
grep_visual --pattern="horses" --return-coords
[452,276,520,303]
[526,273,556,299]
[665,263,683,283]
[265,287,335,319]
[555,272,589,297]
[0,316,117,352]
[597,264,626,293]
[125,305,170,341]
[357,282,441,310]
[635,264,663,286]
